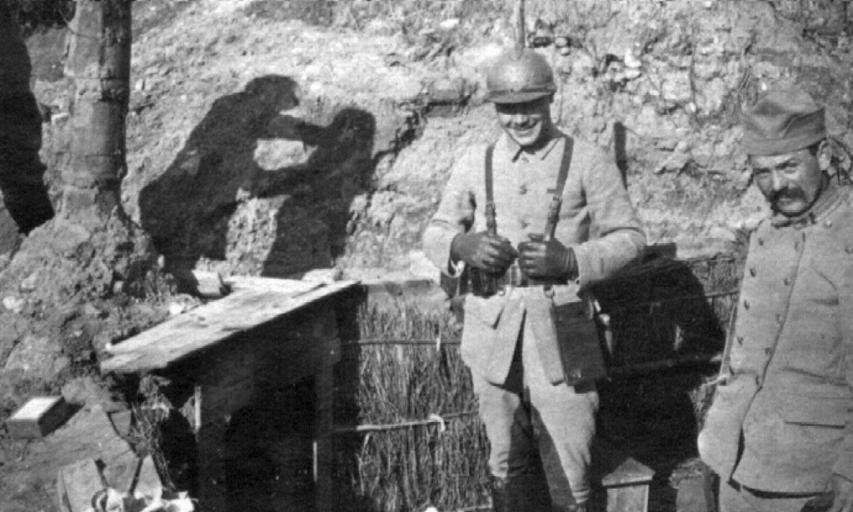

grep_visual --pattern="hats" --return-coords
[742,88,827,156]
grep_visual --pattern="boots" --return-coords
[491,487,530,512]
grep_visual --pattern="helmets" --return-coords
[485,49,556,104]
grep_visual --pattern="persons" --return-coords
[438,42,645,512]
[695,83,852,511]
[1,12,54,233]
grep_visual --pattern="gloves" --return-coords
[452,232,517,276]
[517,233,577,280]
